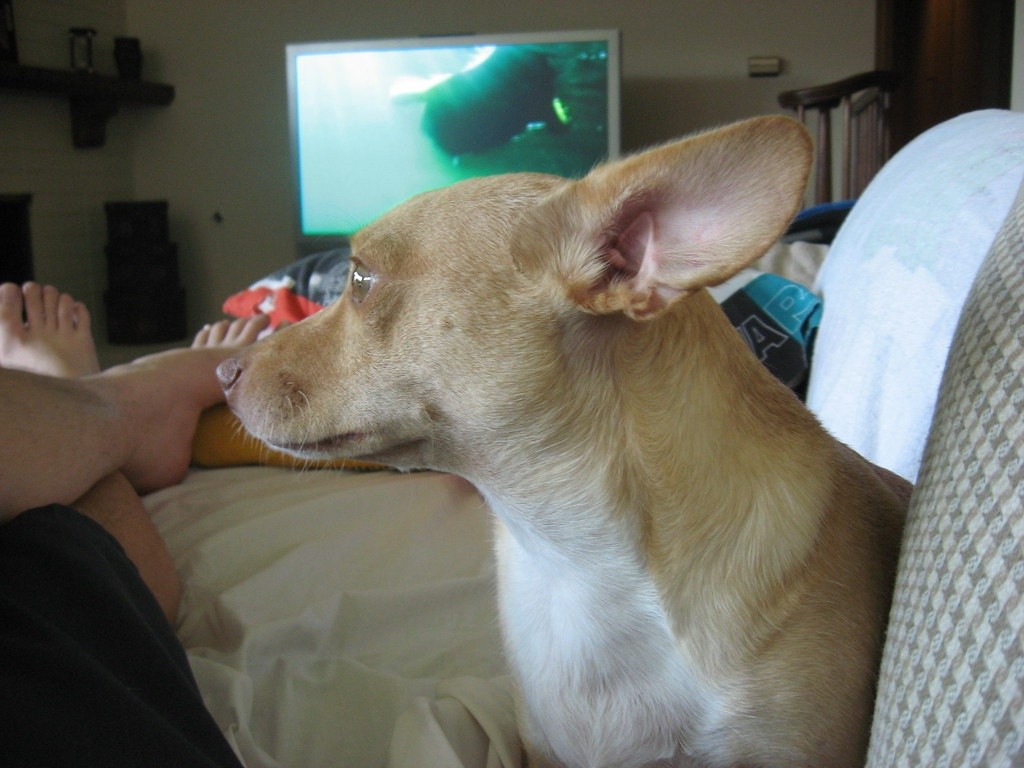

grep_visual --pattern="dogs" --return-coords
[212,115,914,768]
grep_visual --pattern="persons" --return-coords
[0,277,299,768]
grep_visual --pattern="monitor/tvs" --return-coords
[286,27,622,254]
